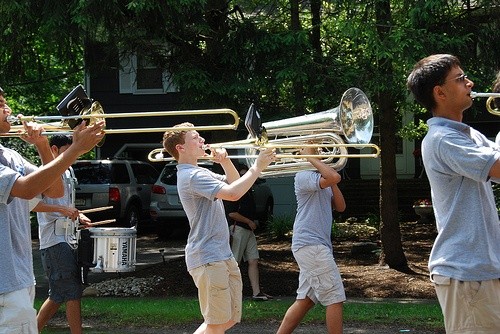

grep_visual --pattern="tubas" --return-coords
[244,86,375,179]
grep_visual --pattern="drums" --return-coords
[78,225,138,274]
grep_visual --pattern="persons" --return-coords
[275,133,347,334]
[28,134,92,334]
[225,163,274,301]
[0,88,105,334]
[162,121,276,334]
[407,54,500,334]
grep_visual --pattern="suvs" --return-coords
[149,159,215,239]
[72,157,160,235]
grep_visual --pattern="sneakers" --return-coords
[253,291,275,301]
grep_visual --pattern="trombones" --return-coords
[1,109,245,139]
[147,125,380,165]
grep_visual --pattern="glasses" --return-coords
[437,73,468,85]
